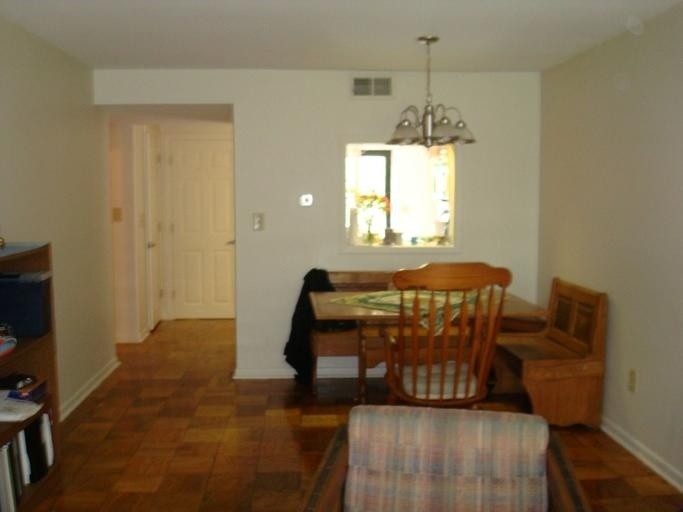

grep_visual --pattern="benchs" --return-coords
[491,277,609,427]
[304,270,469,399]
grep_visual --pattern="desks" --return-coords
[309,290,550,408]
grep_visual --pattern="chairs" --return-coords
[294,406,591,511]
[383,261,512,406]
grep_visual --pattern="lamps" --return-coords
[384,35,476,149]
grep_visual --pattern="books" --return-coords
[0,413,56,512]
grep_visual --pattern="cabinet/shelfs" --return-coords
[0,242,68,512]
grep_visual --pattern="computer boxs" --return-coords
[0,278,52,338]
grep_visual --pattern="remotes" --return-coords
[0,272,20,278]
